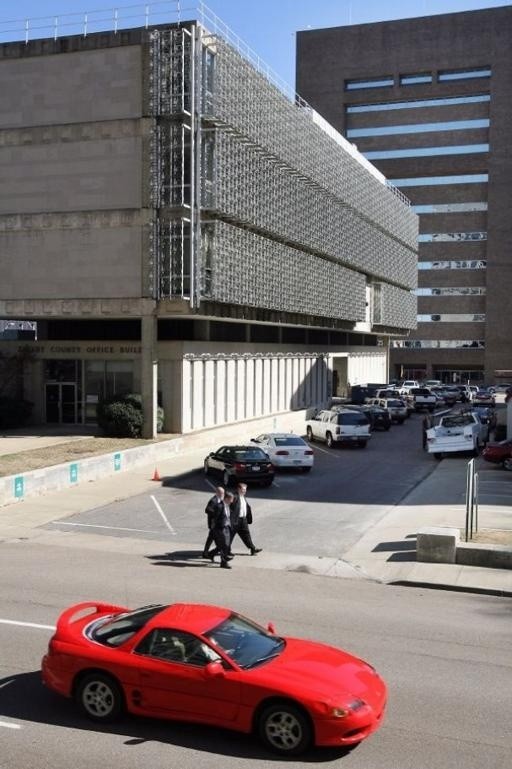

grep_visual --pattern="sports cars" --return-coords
[39,596,392,758]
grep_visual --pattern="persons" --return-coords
[202,482,263,569]
[423,415,432,448]
[490,411,496,431]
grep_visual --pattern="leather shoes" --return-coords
[251,548,263,555]
[202,549,235,569]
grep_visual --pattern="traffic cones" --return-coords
[150,467,161,482]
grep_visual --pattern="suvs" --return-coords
[302,405,374,449]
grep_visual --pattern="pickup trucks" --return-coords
[424,411,491,458]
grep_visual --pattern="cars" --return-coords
[479,437,512,472]
[250,430,315,475]
[338,378,497,431]
[201,444,276,491]
[487,382,512,393]
